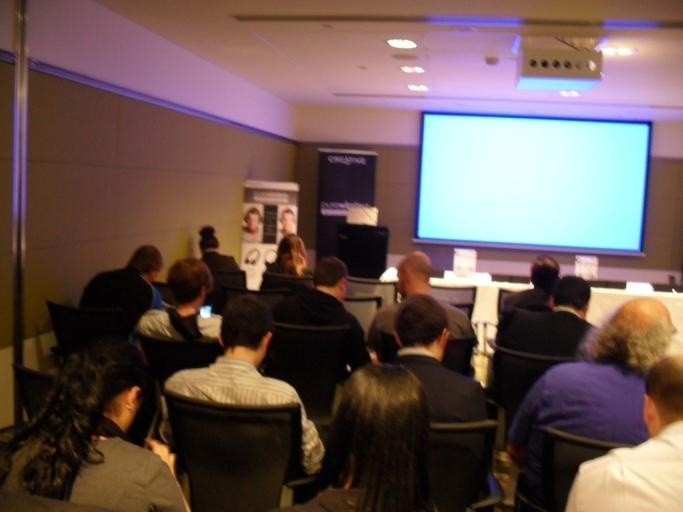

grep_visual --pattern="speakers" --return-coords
[336,222,389,279]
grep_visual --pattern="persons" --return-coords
[365,249,476,365]
[278,208,296,238]
[76,244,163,320]
[0,329,191,511]
[485,255,682,512]
[164,294,326,477]
[241,206,263,242]
[270,255,365,357]
[268,363,437,511]
[129,256,223,366]
[259,233,318,290]
[385,294,492,511]
[199,226,240,273]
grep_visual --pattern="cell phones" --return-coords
[200,305,212,317]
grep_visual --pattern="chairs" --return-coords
[429,285,477,322]
[133,330,223,450]
[345,277,398,307]
[372,336,476,376]
[492,343,580,448]
[271,319,352,415]
[337,295,383,343]
[220,285,290,318]
[0,491,120,512]
[430,420,498,510]
[164,389,319,511]
[46,300,79,352]
[539,425,640,512]
[12,365,56,428]
[211,271,246,293]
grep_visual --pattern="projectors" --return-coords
[514,48,602,91]
